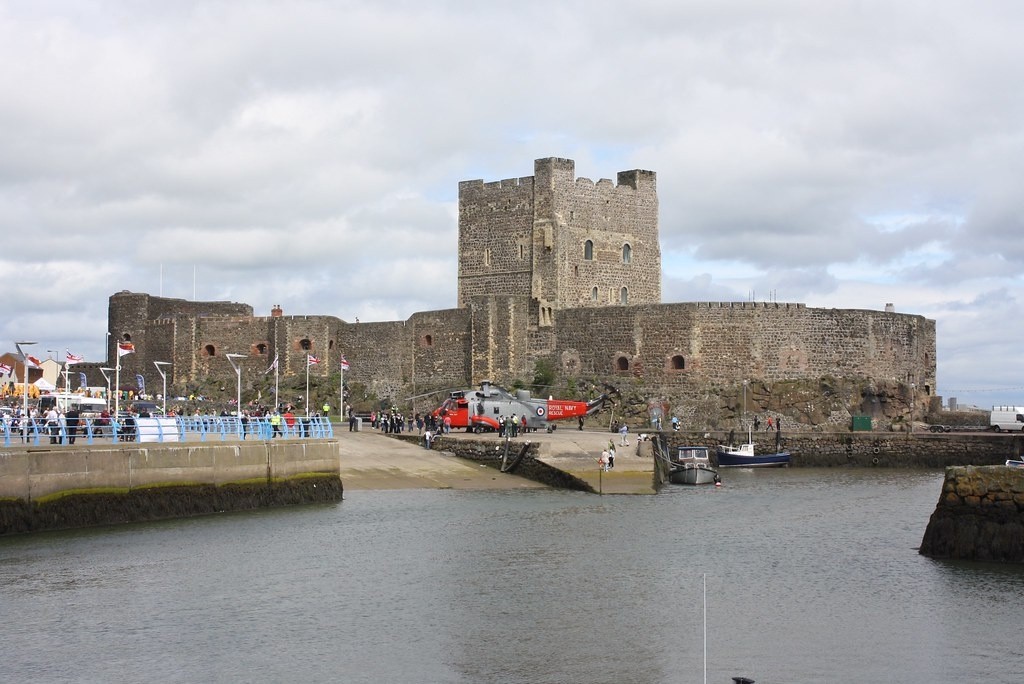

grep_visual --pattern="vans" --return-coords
[990,406,1024,433]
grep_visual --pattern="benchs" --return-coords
[101,426,122,440]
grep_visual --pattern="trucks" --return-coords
[38,391,107,431]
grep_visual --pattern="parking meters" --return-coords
[60,371,75,393]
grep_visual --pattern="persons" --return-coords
[776,415,781,430]
[499,413,519,437]
[520,414,527,427]
[672,415,678,431]
[656,416,662,431]
[602,438,615,472]
[57,386,62,393]
[74,387,116,400]
[241,398,295,439]
[766,416,774,431]
[3,382,40,400]
[0,401,150,445]
[371,411,451,435]
[189,393,205,400]
[118,388,162,400]
[323,404,330,417]
[423,427,441,450]
[349,415,356,431]
[578,415,585,431]
[172,405,228,431]
[611,419,629,447]
[754,415,759,430]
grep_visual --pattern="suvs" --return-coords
[131,402,163,417]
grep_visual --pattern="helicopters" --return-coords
[403,379,624,434]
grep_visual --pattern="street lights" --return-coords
[225,352,248,418]
[153,361,172,418]
[106,331,112,366]
[47,350,58,390]
[911,382,915,433]
[101,367,115,417]
[15,341,40,444]
[742,379,747,432]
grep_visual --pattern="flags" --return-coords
[28,356,40,369]
[119,342,136,356]
[342,356,349,370]
[0,362,11,374]
[66,351,83,364]
[308,355,321,365]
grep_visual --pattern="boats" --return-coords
[715,424,791,468]
[668,445,722,486]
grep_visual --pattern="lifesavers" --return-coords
[847,444,852,451]
[848,452,853,457]
[874,447,880,454]
[873,458,878,464]
